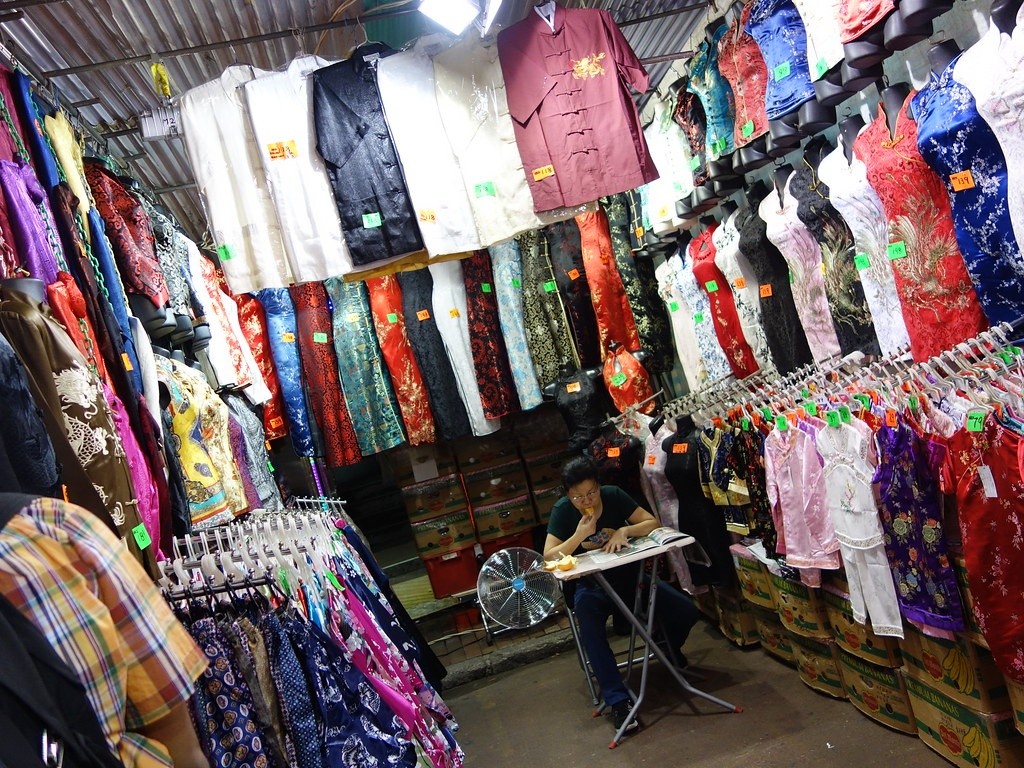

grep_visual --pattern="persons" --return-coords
[586,413,737,596]
[542,456,695,736]
[0,492,215,768]
[640,1,1024,392]
[0,154,284,530]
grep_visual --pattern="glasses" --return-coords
[570,488,597,501]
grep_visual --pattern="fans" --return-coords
[475,547,569,646]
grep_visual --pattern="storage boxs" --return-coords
[389,408,578,599]
[693,543,1024,768]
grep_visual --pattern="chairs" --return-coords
[556,579,680,707]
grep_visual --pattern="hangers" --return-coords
[287,25,320,76]
[536,0,551,7]
[228,40,256,90]
[356,15,381,51]
[658,316,1024,432]
[157,494,345,619]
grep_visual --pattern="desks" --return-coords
[547,537,743,750]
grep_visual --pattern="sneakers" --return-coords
[649,632,689,671]
[612,698,640,735]
